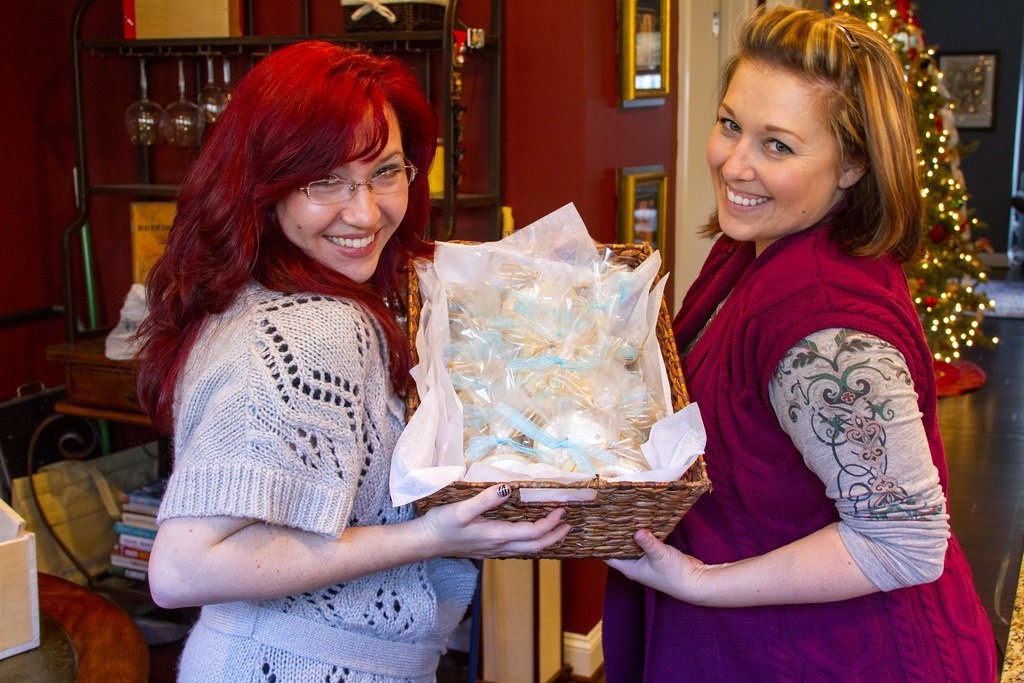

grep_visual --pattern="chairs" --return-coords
[26,412,202,657]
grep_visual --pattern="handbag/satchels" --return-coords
[10,412,161,589]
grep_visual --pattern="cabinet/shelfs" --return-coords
[60,0,509,344]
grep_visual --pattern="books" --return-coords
[110,473,168,585]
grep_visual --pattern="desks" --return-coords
[0,569,150,683]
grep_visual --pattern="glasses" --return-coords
[296,156,415,205]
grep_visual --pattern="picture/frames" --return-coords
[935,46,1002,132]
[626,172,669,266]
[623,0,671,100]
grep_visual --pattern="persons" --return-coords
[600,3,1000,682]
[133,38,572,683]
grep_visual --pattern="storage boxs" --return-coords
[0,498,42,660]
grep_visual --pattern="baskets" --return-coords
[404,240,712,564]
